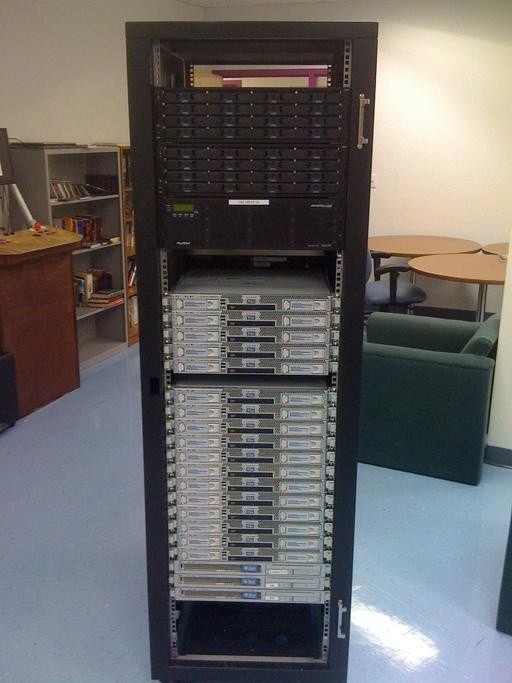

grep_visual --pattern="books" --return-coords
[49,152,136,311]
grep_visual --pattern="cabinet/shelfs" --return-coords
[94,140,139,347]
[10,142,129,366]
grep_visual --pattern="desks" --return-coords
[367,234,510,323]
[0,224,84,419]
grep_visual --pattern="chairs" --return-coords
[360,311,501,487]
